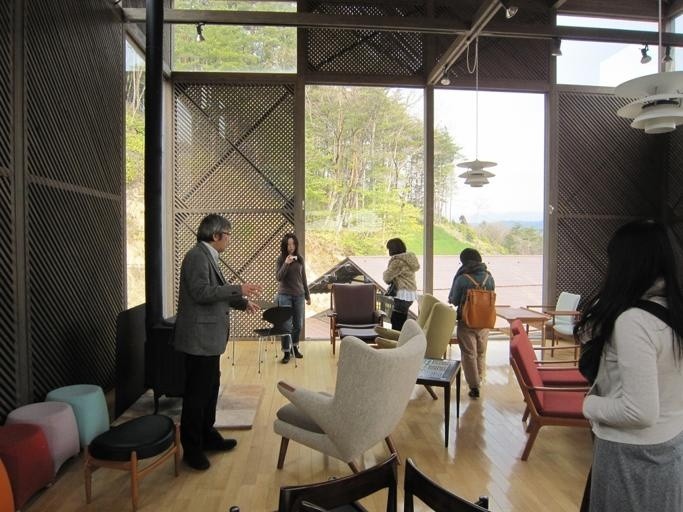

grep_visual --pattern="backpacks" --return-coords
[462,273,496,329]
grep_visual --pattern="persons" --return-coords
[275,232,312,364]
[383,238,420,331]
[574,219,683,512]
[447,248,497,399]
[172,214,263,471]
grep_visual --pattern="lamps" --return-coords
[441,73,450,85]
[661,47,673,64]
[551,37,562,57]
[500,0,519,18]
[615,0,683,134]
[641,49,651,64]
[196,23,206,43]
[455,38,497,189]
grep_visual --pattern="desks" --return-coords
[494,304,552,366]
[416,355,462,447]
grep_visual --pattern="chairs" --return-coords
[375,302,456,408]
[229,452,397,512]
[526,292,581,357]
[510,321,581,363]
[509,333,594,462]
[551,312,586,366]
[329,283,388,355]
[273,319,427,474]
[254,306,297,373]
[403,456,488,512]
[375,294,439,341]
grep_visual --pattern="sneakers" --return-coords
[291,346,303,358]
[282,352,289,363]
[469,391,478,396]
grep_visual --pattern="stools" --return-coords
[46,384,111,446]
[0,423,53,512]
[5,401,80,476]
[0,460,15,511]
[85,414,181,511]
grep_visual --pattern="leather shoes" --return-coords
[204,430,237,451]
[182,450,210,470]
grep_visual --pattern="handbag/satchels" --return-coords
[386,281,396,297]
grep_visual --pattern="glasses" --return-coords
[218,230,231,235]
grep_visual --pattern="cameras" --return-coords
[290,256,298,261]
